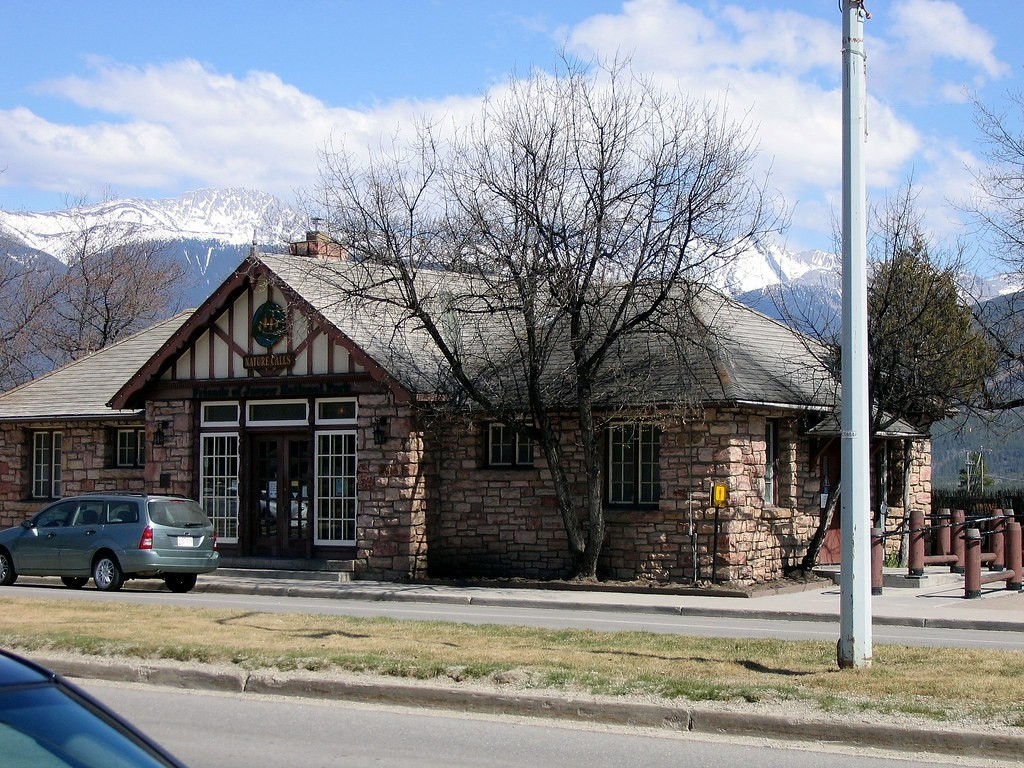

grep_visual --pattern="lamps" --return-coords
[153,421,169,447]
[370,417,388,447]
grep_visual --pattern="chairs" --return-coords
[82,511,98,524]
[117,510,131,521]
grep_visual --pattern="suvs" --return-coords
[0,490,219,592]
